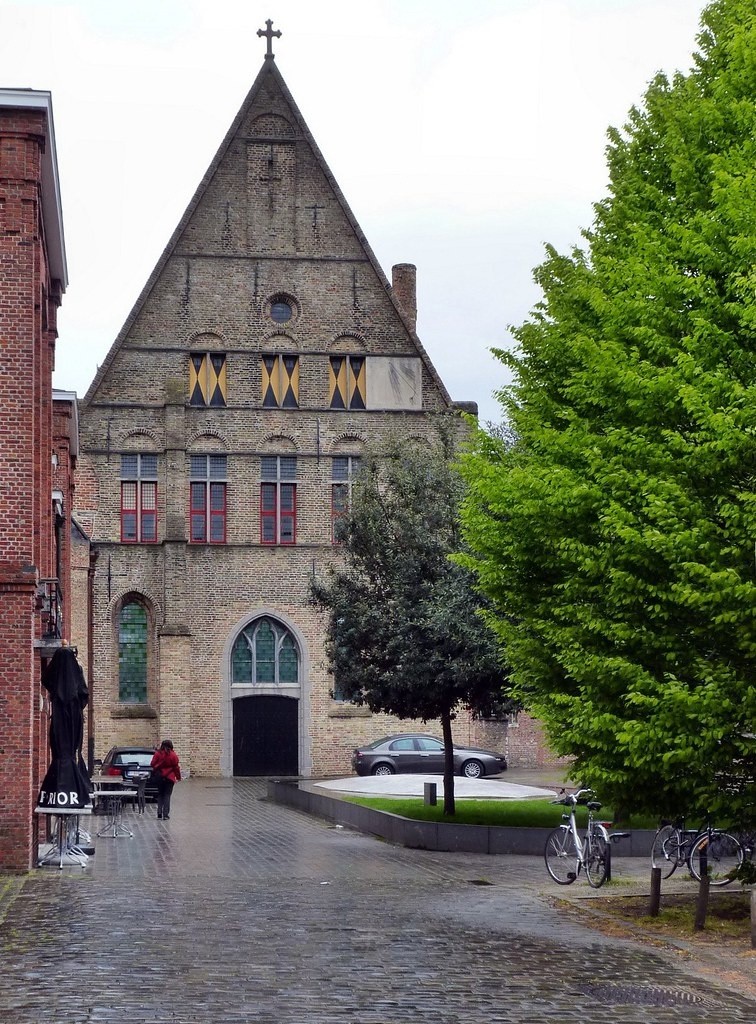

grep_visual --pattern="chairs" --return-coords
[121,776,148,814]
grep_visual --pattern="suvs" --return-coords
[93,746,163,802]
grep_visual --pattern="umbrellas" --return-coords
[40,647,89,807]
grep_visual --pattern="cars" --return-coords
[350,733,509,780]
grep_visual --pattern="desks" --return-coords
[43,805,94,860]
[50,793,95,844]
[91,778,122,815]
[95,791,138,839]
[92,774,123,809]
[35,808,92,871]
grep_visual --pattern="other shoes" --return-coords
[164,816,169,820]
[158,814,162,818]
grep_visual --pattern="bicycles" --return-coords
[650,811,747,886]
[544,788,613,890]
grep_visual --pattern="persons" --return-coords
[150,740,182,820]
